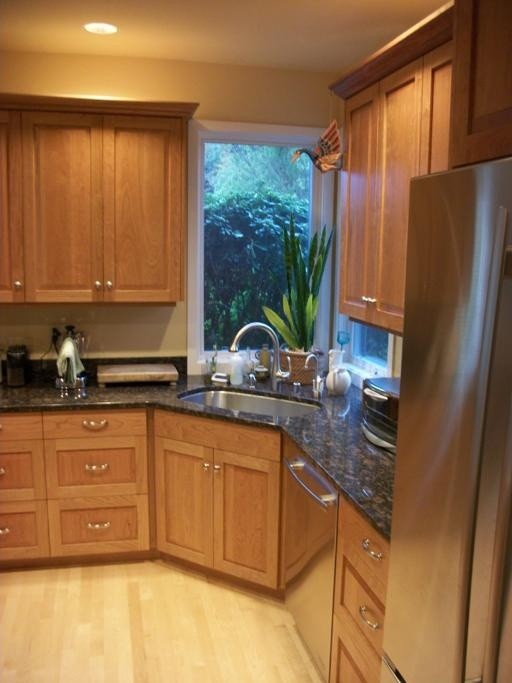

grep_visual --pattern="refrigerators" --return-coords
[381,158,512,677]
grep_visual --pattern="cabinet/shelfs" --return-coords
[25,93,199,302]
[326,0,512,345]
[0,402,153,572]
[150,409,337,603]
[0,91,26,303]
[326,490,391,683]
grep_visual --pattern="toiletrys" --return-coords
[229,346,244,385]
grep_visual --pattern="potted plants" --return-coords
[259,211,335,385]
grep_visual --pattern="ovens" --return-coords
[361,376,404,457]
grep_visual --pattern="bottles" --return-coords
[260,344,270,368]
[327,350,351,397]
[216,346,243,384]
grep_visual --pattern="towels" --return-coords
[56,337,86,389]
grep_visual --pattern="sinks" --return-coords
[179,385,324,417]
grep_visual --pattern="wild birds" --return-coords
[290,118,343,173]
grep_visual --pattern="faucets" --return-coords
[228,321,291,382]
[304,354,322,398]
